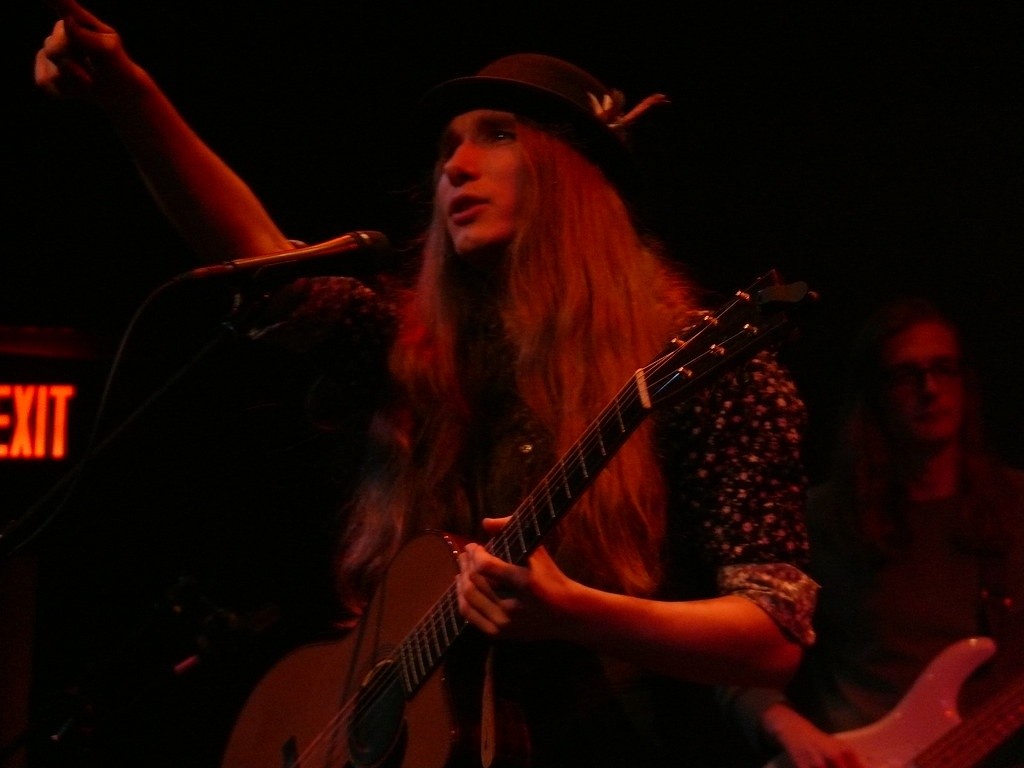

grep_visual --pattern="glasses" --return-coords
[878,353,968,377]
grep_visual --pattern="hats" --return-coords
[416,51,671,192]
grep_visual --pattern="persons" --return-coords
[34,2,822,768]
[738,301,1024,768]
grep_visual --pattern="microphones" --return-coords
[189,231,389,279]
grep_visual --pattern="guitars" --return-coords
[760,636,1024,767]
[215,263,814,768]
